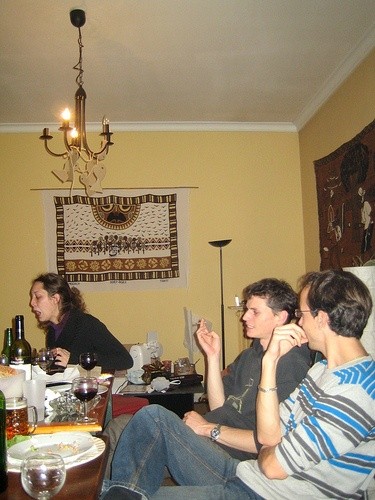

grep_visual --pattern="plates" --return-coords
[46,384,108,395]
[7,433,105,473]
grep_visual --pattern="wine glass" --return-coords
[36,346,57,374]
[79,352,97,377]
[72,377,98,424]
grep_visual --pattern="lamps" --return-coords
[41,10,116,195]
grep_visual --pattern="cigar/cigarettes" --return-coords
[192,321,206,326]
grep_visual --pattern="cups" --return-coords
[21,378,46,421]
[9,365,32,380]
[31,348,37,365]
[20,453,66,500]
[5,397,37,440]
[150,357,195,376]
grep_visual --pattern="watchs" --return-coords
[210,424,221,442]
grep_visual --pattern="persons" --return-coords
[100,269,375,500]
[30,272,134,375]
[102,278,317,480]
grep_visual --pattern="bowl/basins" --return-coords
[0,368,25,402]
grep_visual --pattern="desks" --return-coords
[0,364,205,500]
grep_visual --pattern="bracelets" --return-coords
[258,385,278,393]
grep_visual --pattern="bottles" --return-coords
[2,328,14,366]
[10,315,31,365]
[0,390,8,494]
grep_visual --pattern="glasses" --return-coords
[294,308,318,318]
